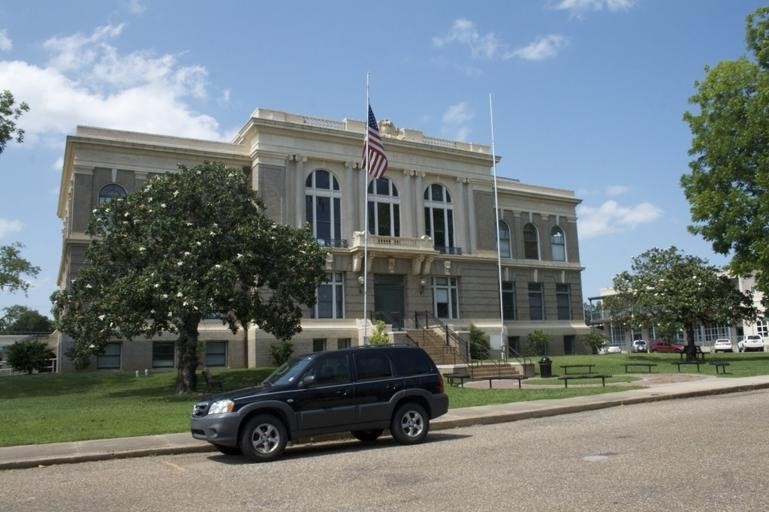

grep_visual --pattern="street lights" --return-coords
[623,313,642,342]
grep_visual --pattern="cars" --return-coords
[714,339,733,353]
[630,340,647,352]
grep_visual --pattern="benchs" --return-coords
[443,345,731,388]
[202,369,225,393]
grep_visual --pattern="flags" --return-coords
[359,104,387,178]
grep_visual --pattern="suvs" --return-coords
[191,342,449,461]
[649,339,685,353]
[738,334,764,352]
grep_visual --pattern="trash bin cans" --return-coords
[538,357,552,377]
